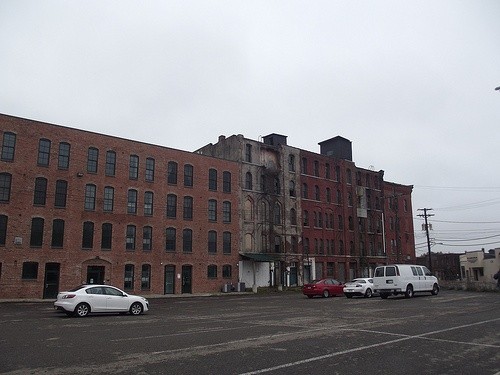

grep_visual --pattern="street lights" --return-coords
[416,208,435,275]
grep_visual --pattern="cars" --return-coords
[343,278,381,298]
[54,284,149,318]
[302,279,347,298]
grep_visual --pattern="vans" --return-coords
[373,264,440,299]
